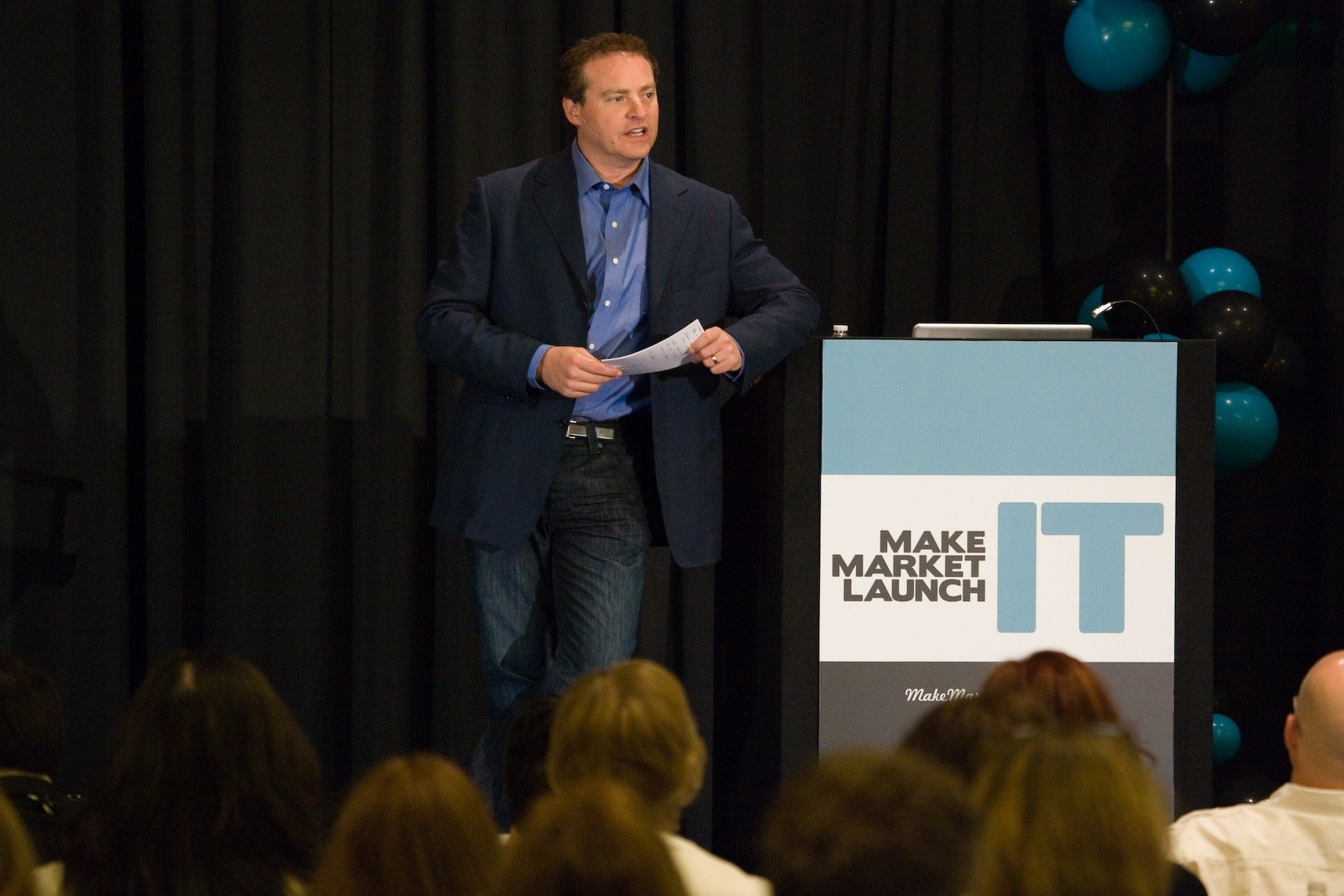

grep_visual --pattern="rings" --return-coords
[711,355,719,367]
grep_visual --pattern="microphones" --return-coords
[1092,300,1163,339]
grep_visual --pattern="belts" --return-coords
[564,420,624,441]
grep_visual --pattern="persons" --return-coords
[416,32,824,829]
[0,630,1344,896]
